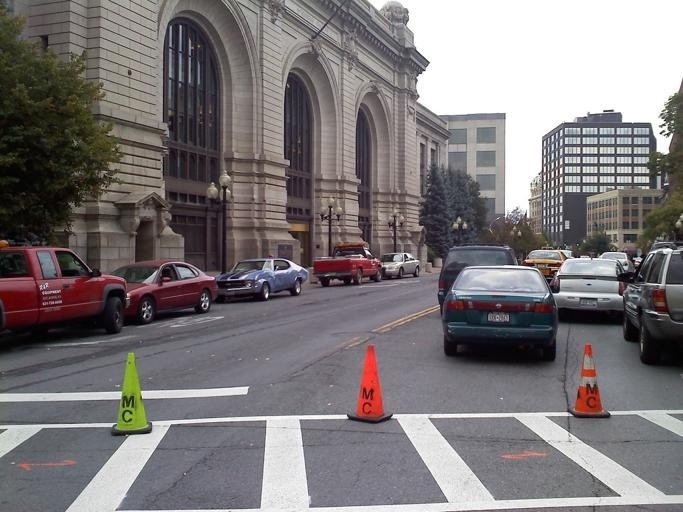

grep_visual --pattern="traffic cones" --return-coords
[569,342,613,417]
[109,351,153,437]
[345,345,394,421]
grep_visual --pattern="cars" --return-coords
[649,239,680,253]
[524,244,567,285]
[552,257,625,321]
[595,249,634,278]
[442,264,561,361]
[436,244,517,314]
[379,251,425,285]
[102,255,218,326]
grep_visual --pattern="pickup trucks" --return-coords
[304,238,385,292]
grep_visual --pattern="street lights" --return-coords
[386,209,405,252]
[317,197,344,254]
[207,167,233,273]
[451,214,468,244]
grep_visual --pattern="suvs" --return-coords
[618,240,682,365]
[0,244,126,332]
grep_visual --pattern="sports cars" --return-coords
[210,252,308,300]
